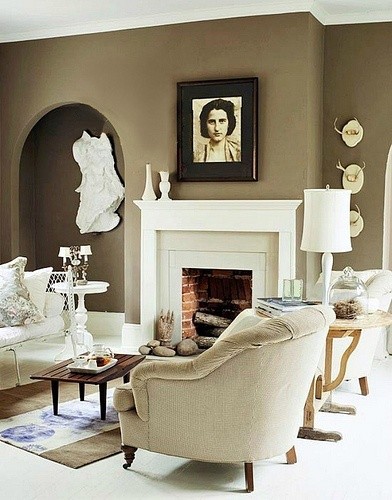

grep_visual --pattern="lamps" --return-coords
[58,245,93,285]
[300,185,352,305]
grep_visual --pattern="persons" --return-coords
[193,99,240,162]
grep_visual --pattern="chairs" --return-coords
[113,306,337,492]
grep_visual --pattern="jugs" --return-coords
[92,343,114,368]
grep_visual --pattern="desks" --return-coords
[133,200,302,333]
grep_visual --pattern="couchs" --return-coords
[313,269,392,395]
[0,292,77,387]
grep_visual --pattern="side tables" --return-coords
[298,309,392,441]
[53,281,110,362]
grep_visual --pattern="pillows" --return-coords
[0,256,53,328]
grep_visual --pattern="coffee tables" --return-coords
[30,350,146,420]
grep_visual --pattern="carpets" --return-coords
[0,381,123,468]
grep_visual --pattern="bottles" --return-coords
[328,266,370,323]
[72,331,90,363]
[157,171,173,200]
[141,163,157,200]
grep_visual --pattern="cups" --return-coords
[88,360,98,368]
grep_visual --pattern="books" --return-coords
[255,298,322,319]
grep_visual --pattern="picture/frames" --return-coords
[176,77,260,182]
